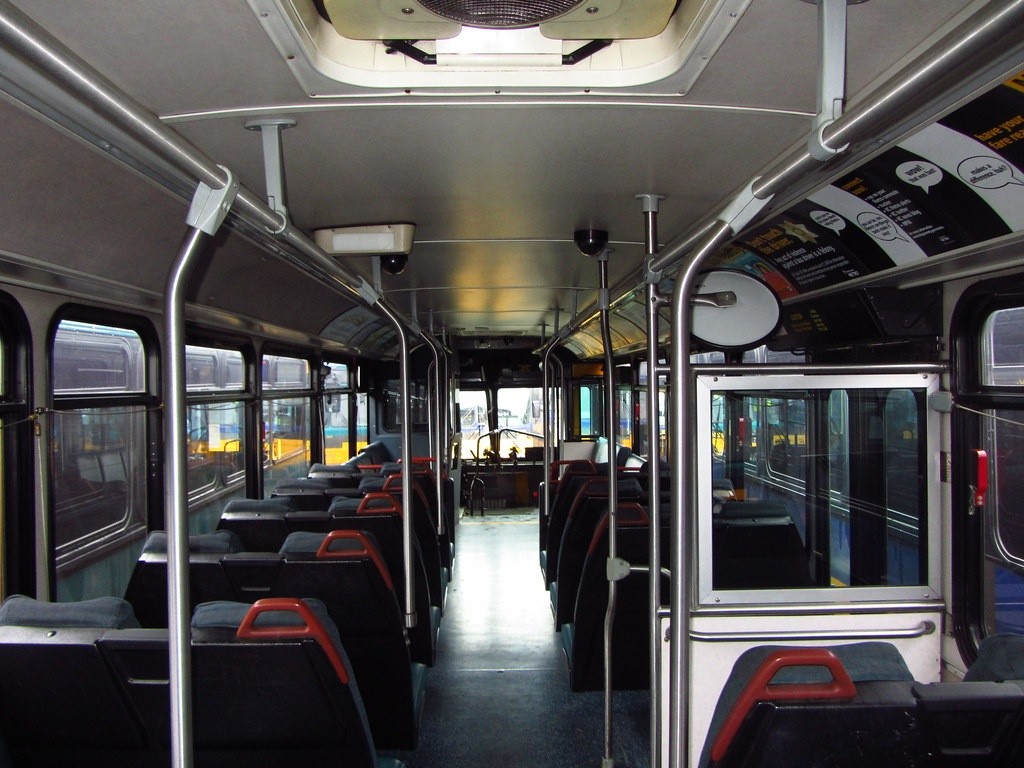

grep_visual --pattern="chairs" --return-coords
[535,459,1024,767]
[0,456,463,768]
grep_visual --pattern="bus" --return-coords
[0,316,373,557]
[451,305,1024,562]
